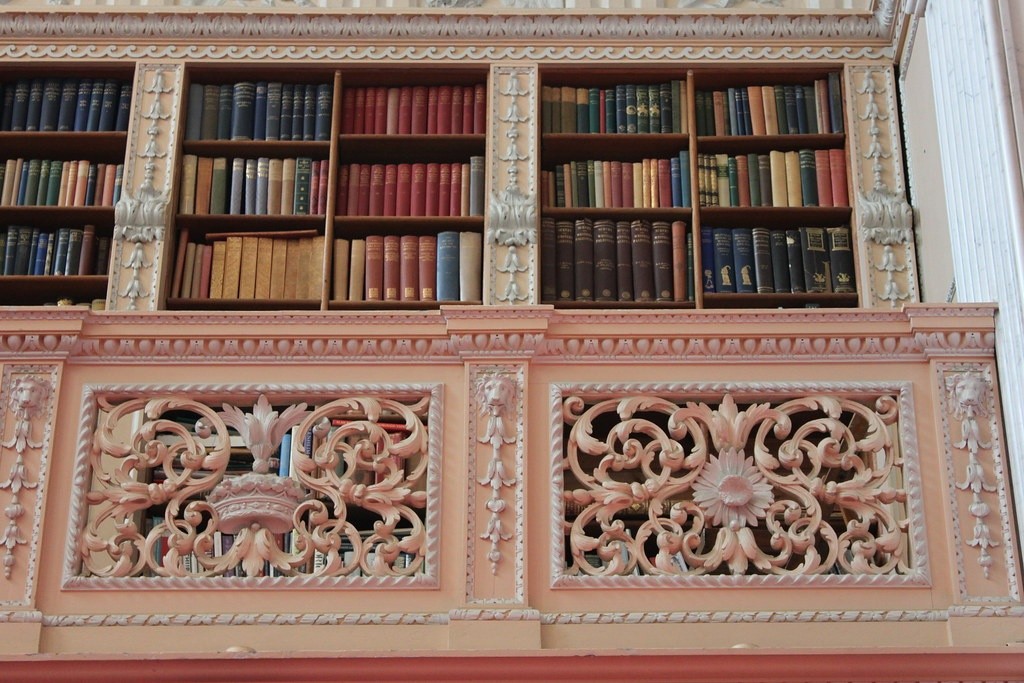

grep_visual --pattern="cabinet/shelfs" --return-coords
[153,58,493,312]
[560,394,911,578]
[123,401,430,576]
[0,61,139,313]
[531,60,870,310]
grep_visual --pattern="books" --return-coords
[46,297,106,312]
[136,408,427,577]
[185,75,486,133]
[174,153,486,215]
[0,77,131,135]
[545,149,848,206]
[1,157,124,208]
[538,78,843,134]
[540,216,856,301]
[170,229,482,301]
[0,224,110,274]
